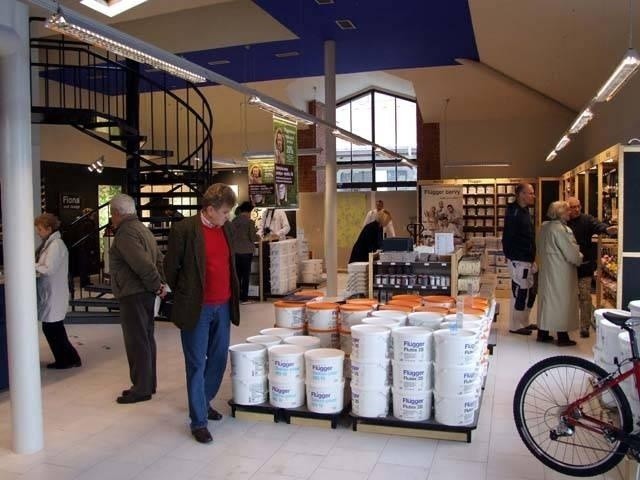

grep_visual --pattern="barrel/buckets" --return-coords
[302,255,325,285]
[376,294,490,345]
[589,300,640,417]
[274,288,379,349]
[230,327,346,415]
[350,310,487,428]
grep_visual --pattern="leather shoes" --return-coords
[117,391,151,403]
[192,427,213,442]
[208,405,222,420]
[510,324,589,346]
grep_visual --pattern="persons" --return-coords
[75,204,184,288]
[164,184,240,443]
[349,210,392,264]
[249,166,261,184]
[227,201,291,303]
[565,197,619,338]
[109,193,167,403]
[275,129,285,164]
[426,201,462,237]
[502,182,538,335]
[33,212,81,369]
[363,200,395,240]
[535,201,584,346]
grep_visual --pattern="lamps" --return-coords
[247,95,316,126]
[87,155,105,174]
[569,108,594,135]
[331,130,418,168]
[593,48,640,103]
[44,13,206,84]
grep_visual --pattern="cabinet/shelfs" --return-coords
[416,178,496,247]
[241,238,278,301]
[561,143,640,329]
[368,247,463,299]
[495,178,539,239]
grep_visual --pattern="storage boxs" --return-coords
[488,249,512,290]
[249,239,323,297]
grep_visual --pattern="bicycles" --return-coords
[512,306,640,476]
